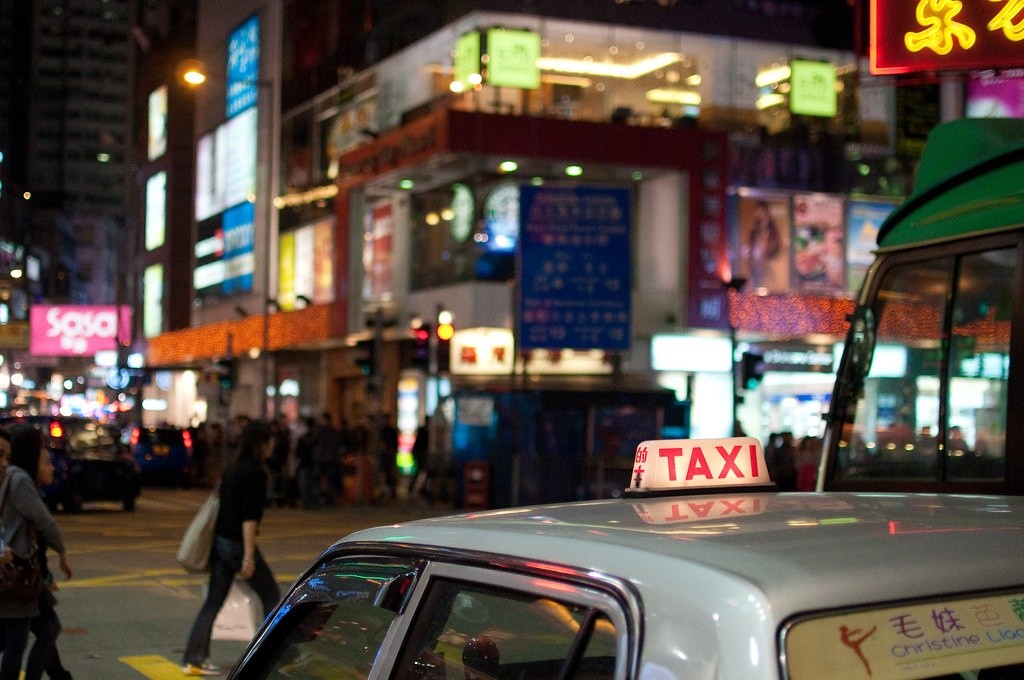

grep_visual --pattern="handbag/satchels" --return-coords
[174,493,220,575]
[0,535,51,602]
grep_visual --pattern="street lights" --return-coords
[182,58,274,420]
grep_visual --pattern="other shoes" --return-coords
[182,657,222,677]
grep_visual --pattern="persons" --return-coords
[180,421,313,675]
[0,424,72,680]
[764,420,996,490]
[40,411,451,521]
[0,429,11,484]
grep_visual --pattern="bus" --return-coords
[810,116,1024,489]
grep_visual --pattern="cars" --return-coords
[1,412,143,521]
[227,435,1024,680]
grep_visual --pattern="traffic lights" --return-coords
[414,322,430,362]
[436,301,456,373]
